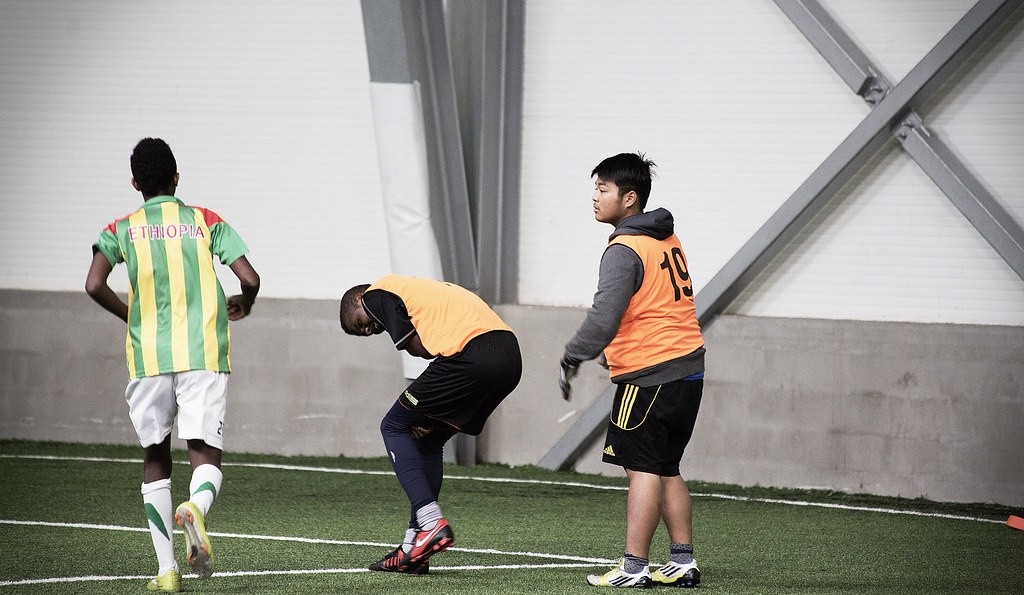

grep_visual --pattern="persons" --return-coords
[85,136,261,592]
[559,153,706,590]
[339,275,522,572]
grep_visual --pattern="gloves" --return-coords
[556,352,583,402]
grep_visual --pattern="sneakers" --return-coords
[146,570,182,593]
[650,558,700,587]
[586,556,653,589]
[396,518,455,573]
[174,501,216,578]
[368,544,429,574]
